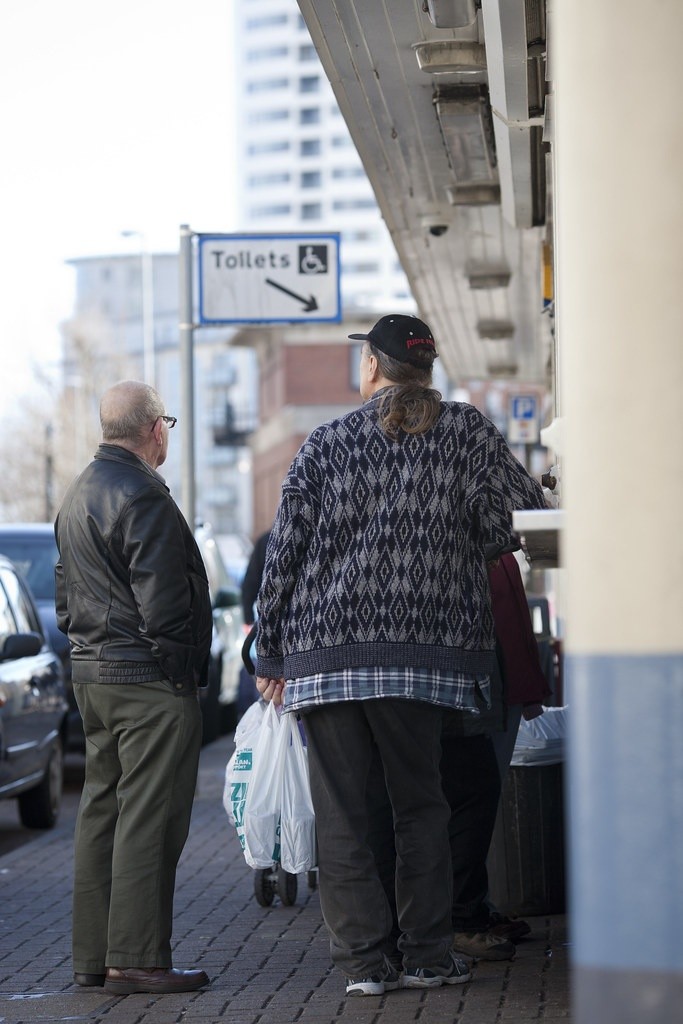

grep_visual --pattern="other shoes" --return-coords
[105,966,210,995]
[488,915,530,943]
[389,951,402,971]
[72,973,105,987]
[452,930,515,960]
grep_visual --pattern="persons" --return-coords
[256,313,548,995]
[239,532,271,625]
[52,383,214,994]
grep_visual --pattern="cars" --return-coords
[0,556,65,832]
[0,522,87,793]
[196,518,256,735]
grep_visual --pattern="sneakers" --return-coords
[346,953,400,997]
[402,947,473,988]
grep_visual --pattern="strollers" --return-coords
[240,622,319,909]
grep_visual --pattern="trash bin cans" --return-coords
[483,707,568,919]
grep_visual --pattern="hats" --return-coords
[348,314,436,367]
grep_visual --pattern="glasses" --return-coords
[151,414,177,432]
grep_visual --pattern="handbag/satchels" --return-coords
[222,695,317,874]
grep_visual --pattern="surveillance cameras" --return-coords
[421,216,452,235]
